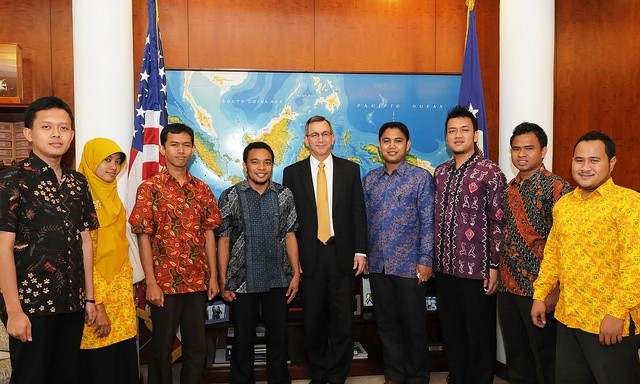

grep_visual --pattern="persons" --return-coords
[75,137,140,384]
[433,104,507,383]
[128,123,223,384]
[498,121,574,383]
[362,120,433,383]
[211,305,223,320]
[215,141,301,384]
[1,96,101,383]
[282,114,368,383]
[531,131,639,382]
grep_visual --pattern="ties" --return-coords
[316,163,331,246]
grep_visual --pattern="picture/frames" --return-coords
[362,275,373,307]
[353,294,361,316]
[204,299,230,324]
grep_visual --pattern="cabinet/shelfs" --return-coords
[198,305,457,383]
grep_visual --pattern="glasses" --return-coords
[306,132,333,140]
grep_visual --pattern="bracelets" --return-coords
[84,299,97,304]
